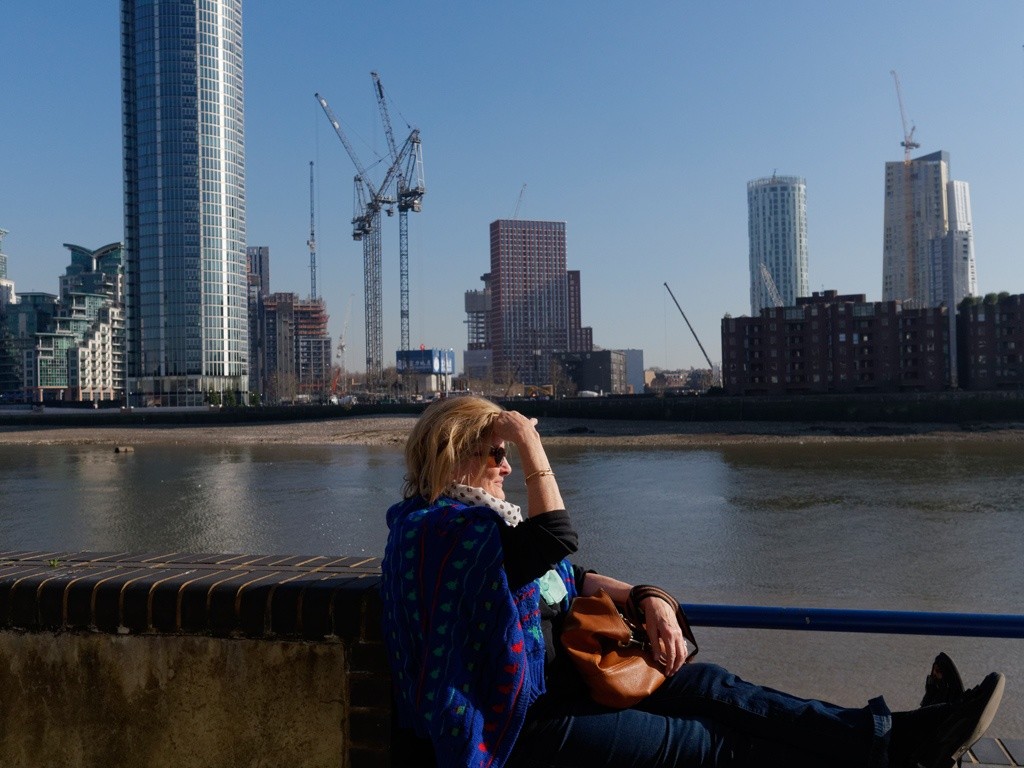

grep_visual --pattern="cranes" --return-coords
[302,69,426,384]
[890,67,922,307]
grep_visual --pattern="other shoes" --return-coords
[917,671,1006,768]
[920,652,965,706]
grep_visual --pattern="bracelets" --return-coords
[525,471,556,480]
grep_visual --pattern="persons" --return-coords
[346,392,1004,768]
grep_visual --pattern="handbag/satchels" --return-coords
[559,585,699,709]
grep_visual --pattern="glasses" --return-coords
[474,447,506,466]
